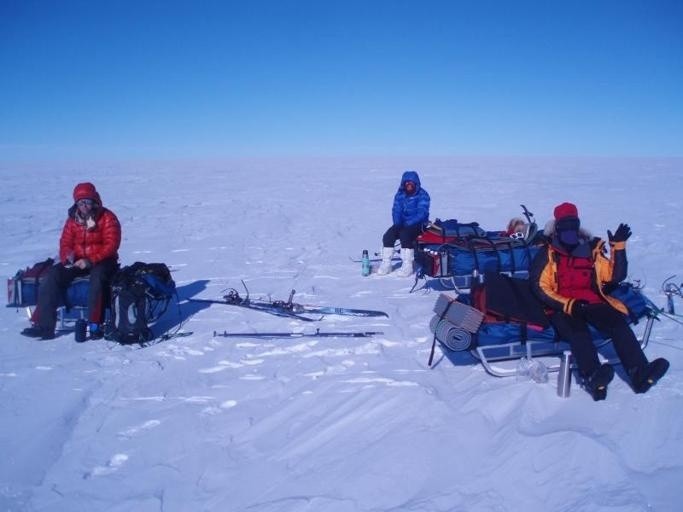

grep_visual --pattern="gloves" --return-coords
[606,222,633,242]
[572,298,591,317]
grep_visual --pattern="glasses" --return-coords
[76,199,94,207]
[510,231,524,241]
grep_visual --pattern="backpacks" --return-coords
[123,262,177,296]
[107,280,152,344]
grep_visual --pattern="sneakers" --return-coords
[586,363,615,402]
[90,323,100,338]
[634,356,669,394]
[23,323,56,339]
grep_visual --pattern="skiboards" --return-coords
[186,278,389,321]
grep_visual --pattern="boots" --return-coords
[377,246,393,275]
[398,247,415,276]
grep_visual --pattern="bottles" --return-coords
[74,318,86,343]
[556,349,572,399]
[361,250,370,277]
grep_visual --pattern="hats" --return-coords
[554,203,578,224]
[73,182,102,206]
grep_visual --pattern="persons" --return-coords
[527,201,669,400]
[19,181,123,340]
[376,171,432,278]
[502,217,525,239]
